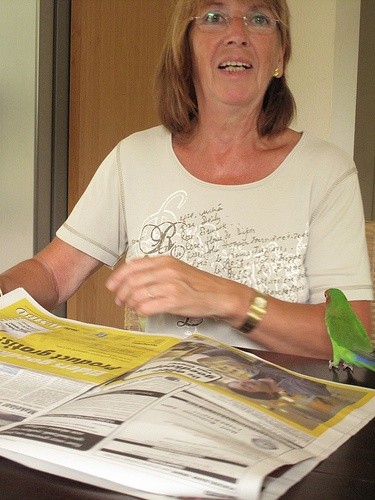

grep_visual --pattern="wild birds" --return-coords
[325,289,375,374]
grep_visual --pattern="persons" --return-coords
[181,348,332,401]
[2,0,374,363]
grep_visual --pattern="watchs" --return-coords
[232,284,268,336]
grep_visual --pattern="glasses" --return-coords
[188,11,280,35]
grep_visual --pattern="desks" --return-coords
[0,343,375,500]
